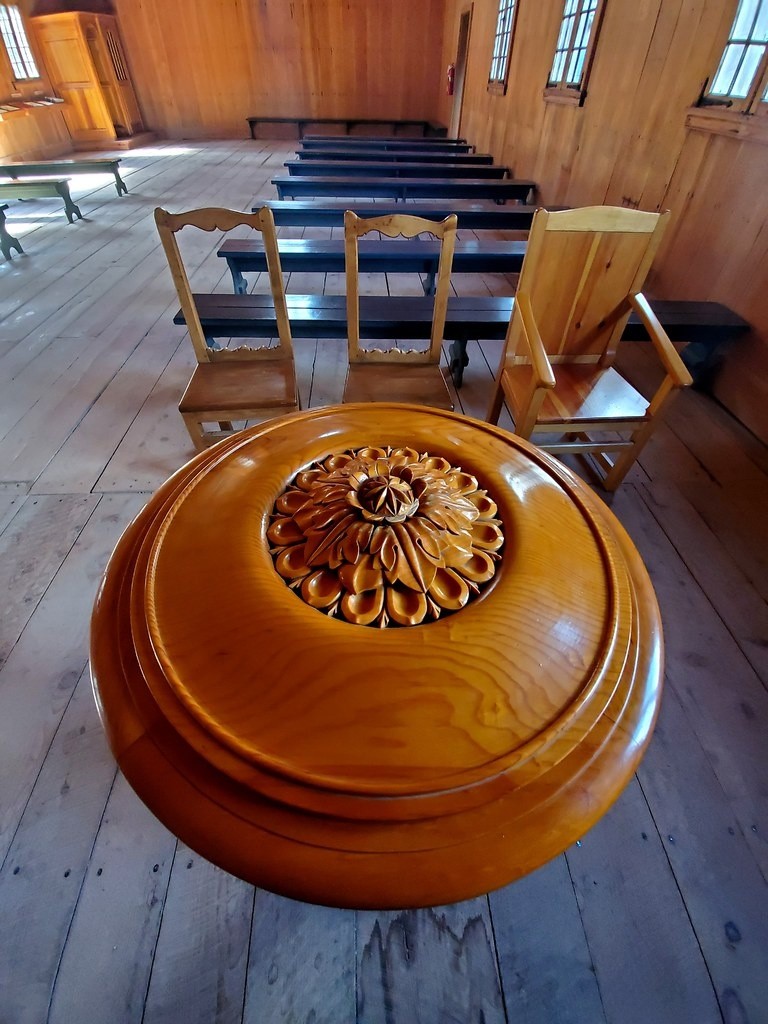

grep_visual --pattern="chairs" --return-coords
[343,210,455,414]
[485,205,694,493]
[155,205,302,455]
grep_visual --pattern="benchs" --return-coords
[0,114,753,397]
